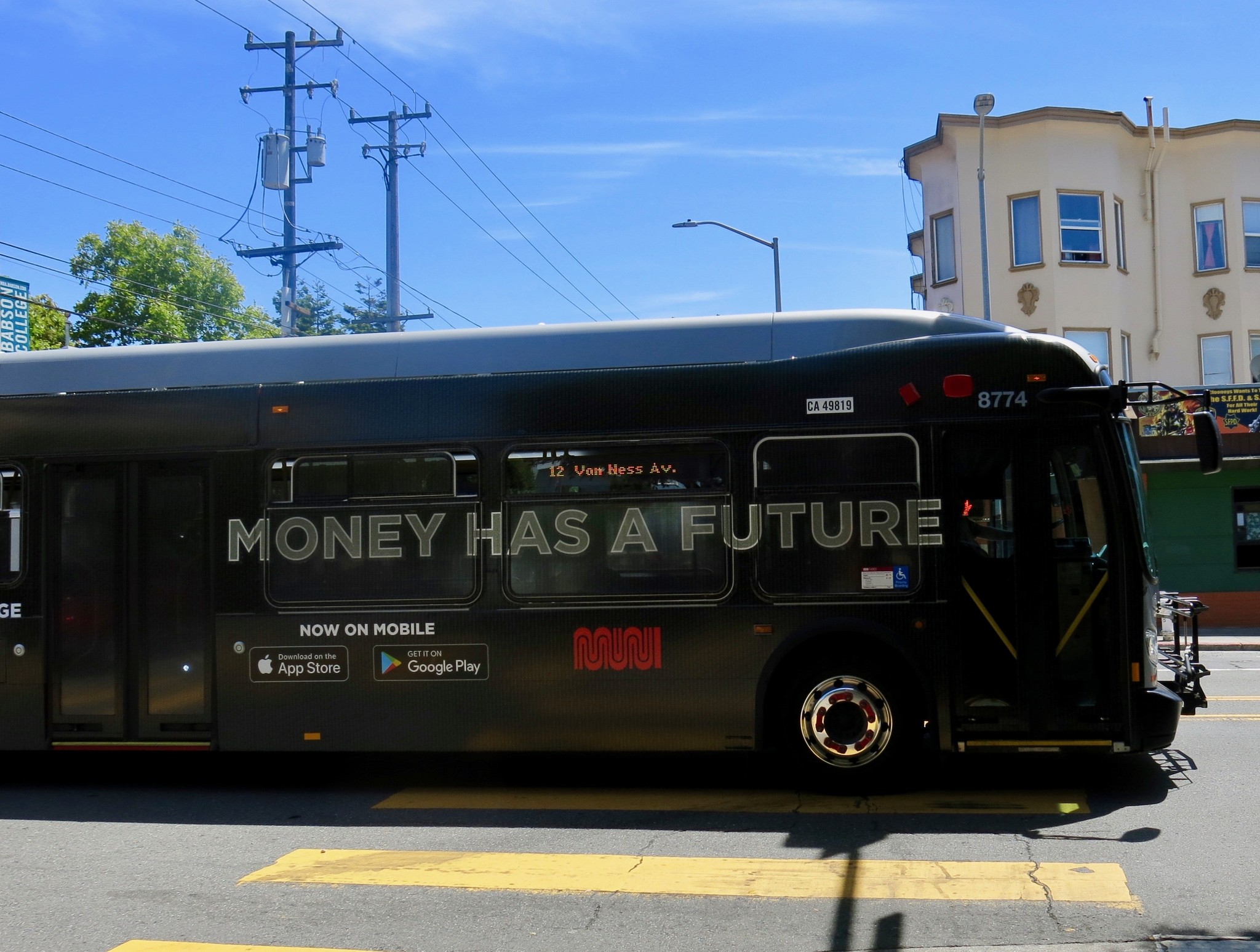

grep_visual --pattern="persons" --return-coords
[959,499,1013,560]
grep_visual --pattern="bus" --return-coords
[0,308,1223,799]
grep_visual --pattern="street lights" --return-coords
[672,217,782,311]
[972,92,996,320]
